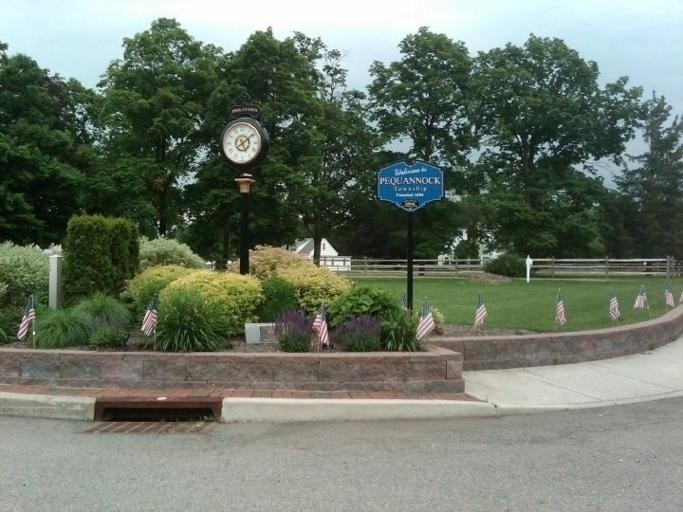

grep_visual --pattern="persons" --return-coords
[437,250,445,266]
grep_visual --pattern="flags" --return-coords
[414,300,435,340]
[312,302,330,348]
[16,296,35,342]
[473,295,487,328]
[666,286,675,307]
[608,290,622,321]
[555,291,567,326]
[632,286,649,311]
[140,296,159,338]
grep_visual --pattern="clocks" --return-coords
[221,116,268,170]
